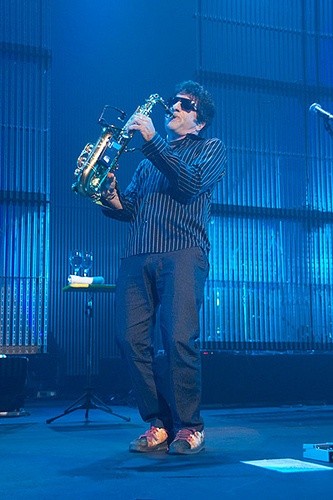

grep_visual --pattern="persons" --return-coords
[89,81,227,453]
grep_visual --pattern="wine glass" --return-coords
[81,252,93,277]
[69,250,82,275]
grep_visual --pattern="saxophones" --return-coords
[70,92,176,210]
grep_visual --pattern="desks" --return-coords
[45,285,131,431]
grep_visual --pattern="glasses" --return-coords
[171,97,201,113]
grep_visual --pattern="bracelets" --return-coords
[106,189,117,199]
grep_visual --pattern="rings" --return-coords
[140,117,143,120]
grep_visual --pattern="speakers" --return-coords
[199,349,333,409]
[0,357,30,413]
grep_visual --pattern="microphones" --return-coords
[309,103,333,119]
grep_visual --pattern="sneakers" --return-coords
[129,426,206,454]
